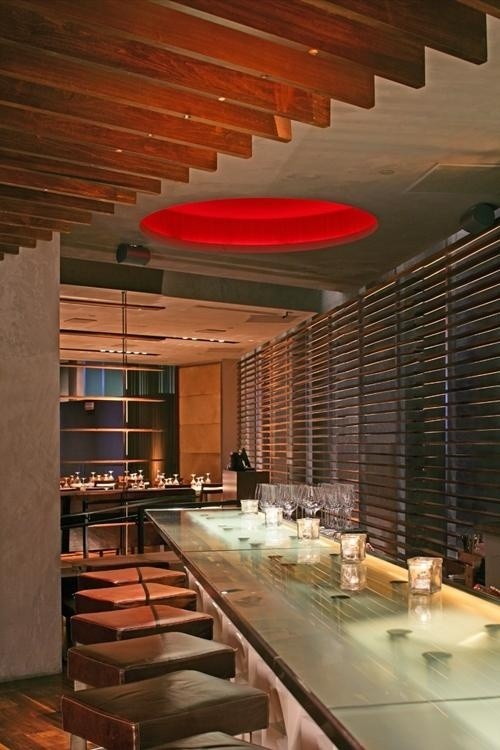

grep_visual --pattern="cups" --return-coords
[240,498,259,515]
[339,533,367,563]
[407,555,444,594]
[341,563,367,592]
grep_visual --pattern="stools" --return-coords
[62,553,270,749]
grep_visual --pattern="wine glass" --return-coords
[253,480,356,540]
[155,470,181,488]
[123,469,144,482]
[90,470,114,482]
[189,472,212,490]
[60,472,88,493]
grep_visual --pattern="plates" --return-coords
[96,484,116,489]
